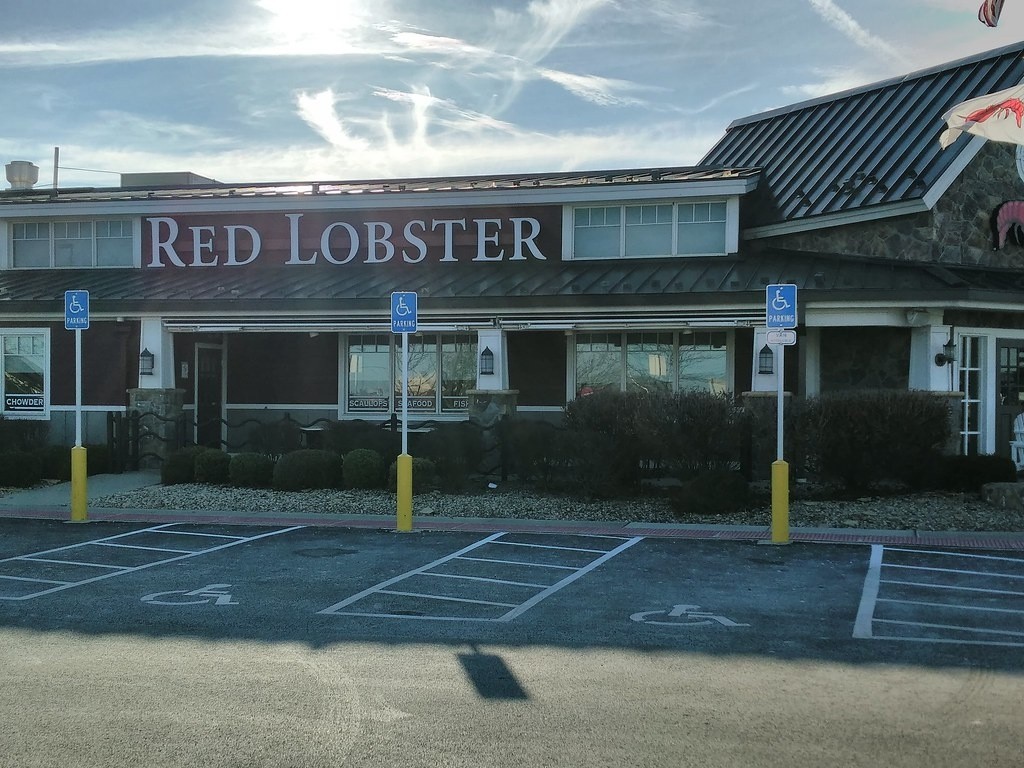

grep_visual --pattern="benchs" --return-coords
[1009,413,1024,475]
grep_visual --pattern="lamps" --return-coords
[480,347,494,375]
[140,348,154,376]
[758,344,774,375]
[935,340,957,366]
